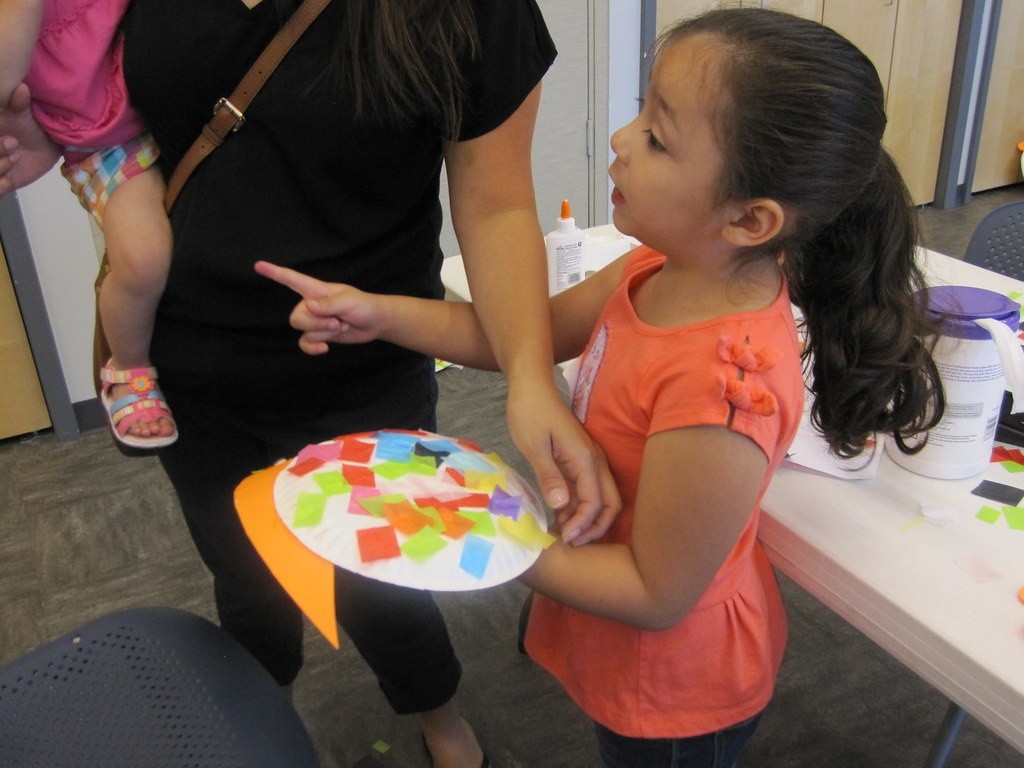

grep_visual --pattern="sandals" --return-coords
[100,357,180,457]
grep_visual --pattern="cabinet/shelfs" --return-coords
[639,0,984,211]
[961,0,1024,205]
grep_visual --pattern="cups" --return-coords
[886,285,1018,479]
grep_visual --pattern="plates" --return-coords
[271,427,550,592]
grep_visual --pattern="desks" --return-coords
[439,223,1023,768]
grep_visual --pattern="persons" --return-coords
[0,0,622,766]
[0,2,183,451]
[255,5,953,768]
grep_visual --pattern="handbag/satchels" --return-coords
[92,247,112,398]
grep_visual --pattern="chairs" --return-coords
[1,604,319,768]
